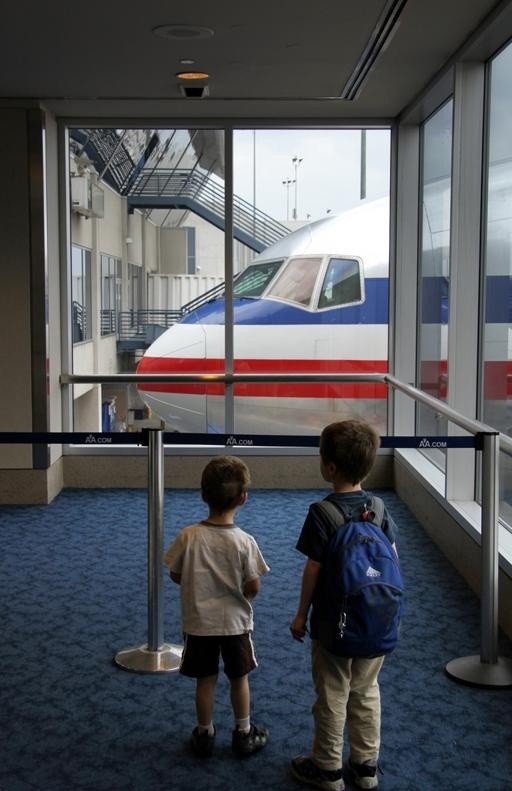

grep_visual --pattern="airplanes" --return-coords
[136,160,512,501]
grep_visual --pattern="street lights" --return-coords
[291,154,306,219]
[281,176,298,219]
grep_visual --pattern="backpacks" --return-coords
[310,496,404,657]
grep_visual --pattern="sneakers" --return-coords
[344,757,379,791]
[192,723,216,759]
[290,754,346,791]
[233,723,270,759]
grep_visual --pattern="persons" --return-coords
[162,456,271,760]
[289,420,399,791]
